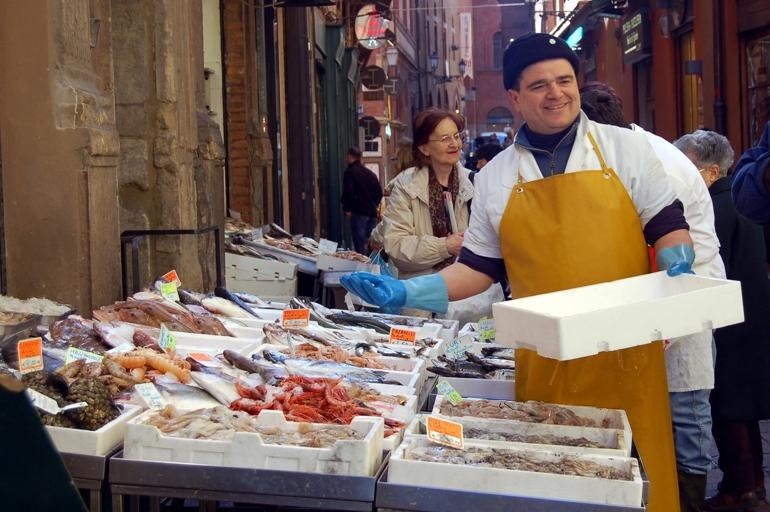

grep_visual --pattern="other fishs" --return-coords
[112,382,224,411]
[290,295,400,334]
[185,355,250,407]
[223,349,258,372]
[426,346,516,380]
[93,274,264,337]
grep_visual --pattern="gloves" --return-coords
[339,270,449,313]
[657,245,696,276]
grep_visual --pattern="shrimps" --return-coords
[51,346,192,394]
[229,374,405,438]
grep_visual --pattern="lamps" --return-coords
[386,41,399,66]
[330,3,390,22]
[345,29,398,42]
[410,52,439,81]
[436,59,466,84]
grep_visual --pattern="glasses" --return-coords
[428,133,464,144]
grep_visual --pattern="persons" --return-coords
[340,77,769,512]
[342,33,695,512]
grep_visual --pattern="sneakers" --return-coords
[704,484,765,511]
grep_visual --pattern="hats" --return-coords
[501,31,579,89]
